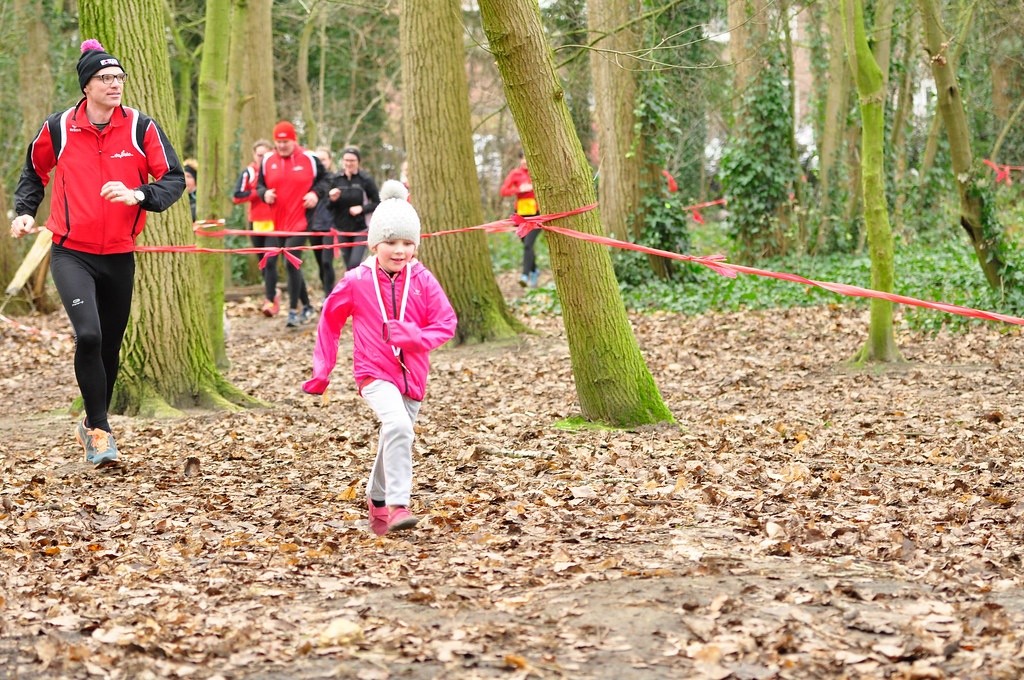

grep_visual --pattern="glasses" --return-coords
[92,74,129,87]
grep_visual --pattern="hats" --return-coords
[76,38,124,93]
[273,121,298,141]
[366,179,420,248]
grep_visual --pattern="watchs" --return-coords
[134,189,145,203]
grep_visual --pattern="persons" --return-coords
[232,140,281,316]
[184,159,196,218]
[307,148,334,298]
[498,150,541,287]
[256,122,328,328]
[302,179,457,537]
[327,146,380,268]
[10,39,187,470]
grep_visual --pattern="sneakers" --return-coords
[74,414,119,467]
[388,505,417,530]
[288,311,300,327]
[366,495,389,536]
[301,307,317,324]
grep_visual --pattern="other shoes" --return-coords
[263,297,279,316]
[519,268,540,286]
